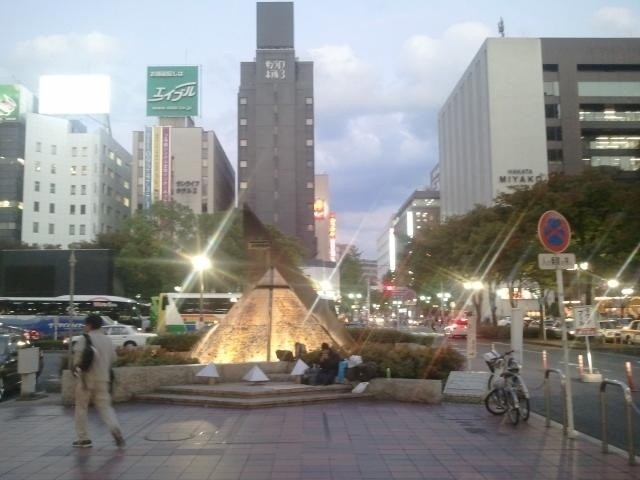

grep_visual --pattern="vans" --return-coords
[444,319,469,339]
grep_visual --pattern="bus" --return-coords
[0,295,143,340]
[157,293,336,335]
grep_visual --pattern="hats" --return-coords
[321,343,328,350]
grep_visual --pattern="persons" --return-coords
[302,342,340,386]
[70,311,127,449]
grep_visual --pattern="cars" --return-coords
[599,319,640,344]
[62,324,158,348]
[529,318,576,338]
[0,322,44,400]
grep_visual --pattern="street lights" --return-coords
[190,255,210,321]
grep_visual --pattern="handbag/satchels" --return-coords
[338,354,376,381]
[78,345,99,372]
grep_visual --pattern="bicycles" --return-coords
[484,350,530,424]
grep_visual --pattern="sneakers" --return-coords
[112,427,125,445]
[72,440,92,447]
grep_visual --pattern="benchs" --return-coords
[264,361,354,383]
